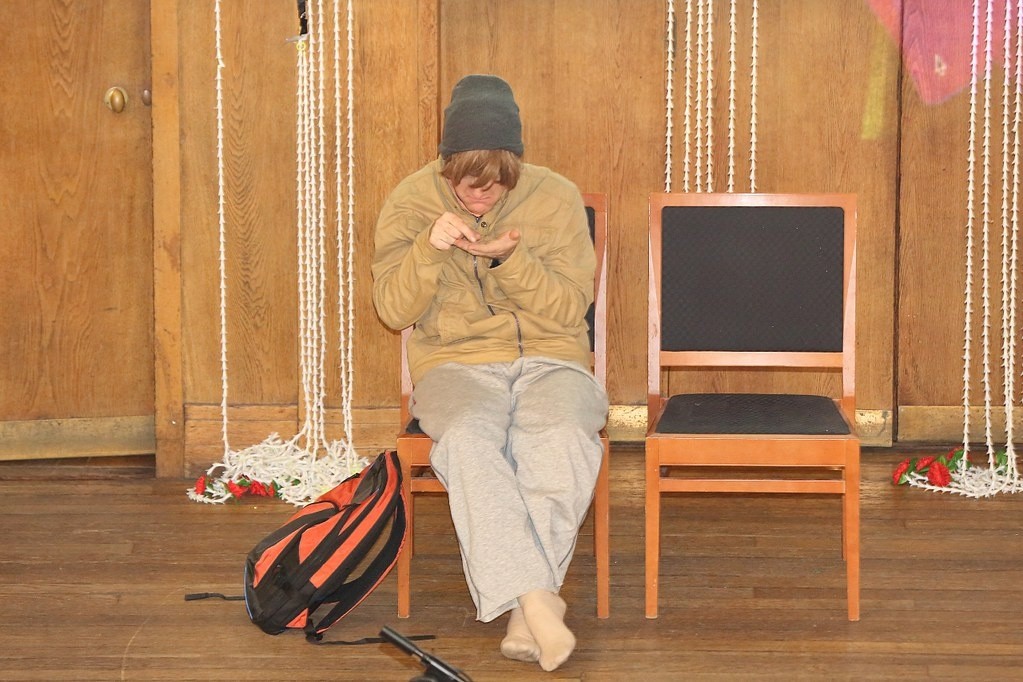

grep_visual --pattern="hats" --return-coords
[438,74,526,157]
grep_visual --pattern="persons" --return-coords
[371,74,608,672]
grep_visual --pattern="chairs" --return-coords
[645,192,863,622]
[393,191,609,619]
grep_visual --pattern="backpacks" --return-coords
[243,449,408,636]
